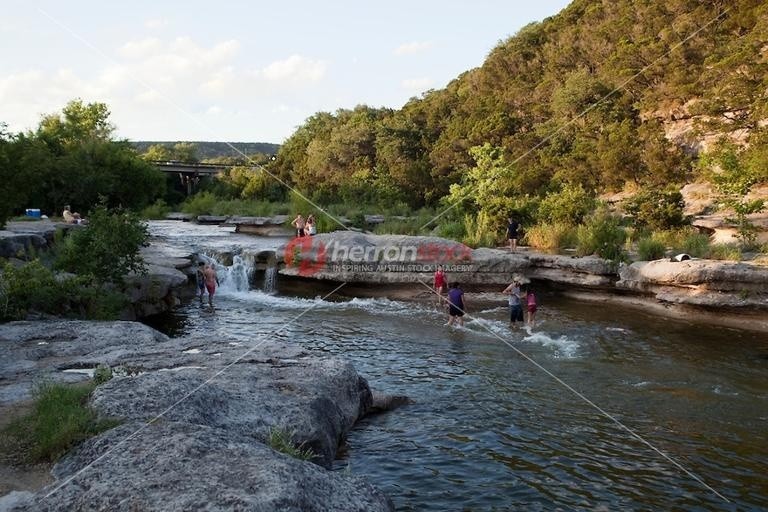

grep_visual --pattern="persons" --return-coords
[202,263,221,309]
[511,286,538,325]
[433,265,450,306]
[308,213,317,227]
[303,218,317,237]
[291,213,306,237]
[443,281,467,328]
[505,215,523,254]
[196,260,210,303]
[502,277,525,329]
[63,205,82,224]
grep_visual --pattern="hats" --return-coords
[514,277,521,282]
